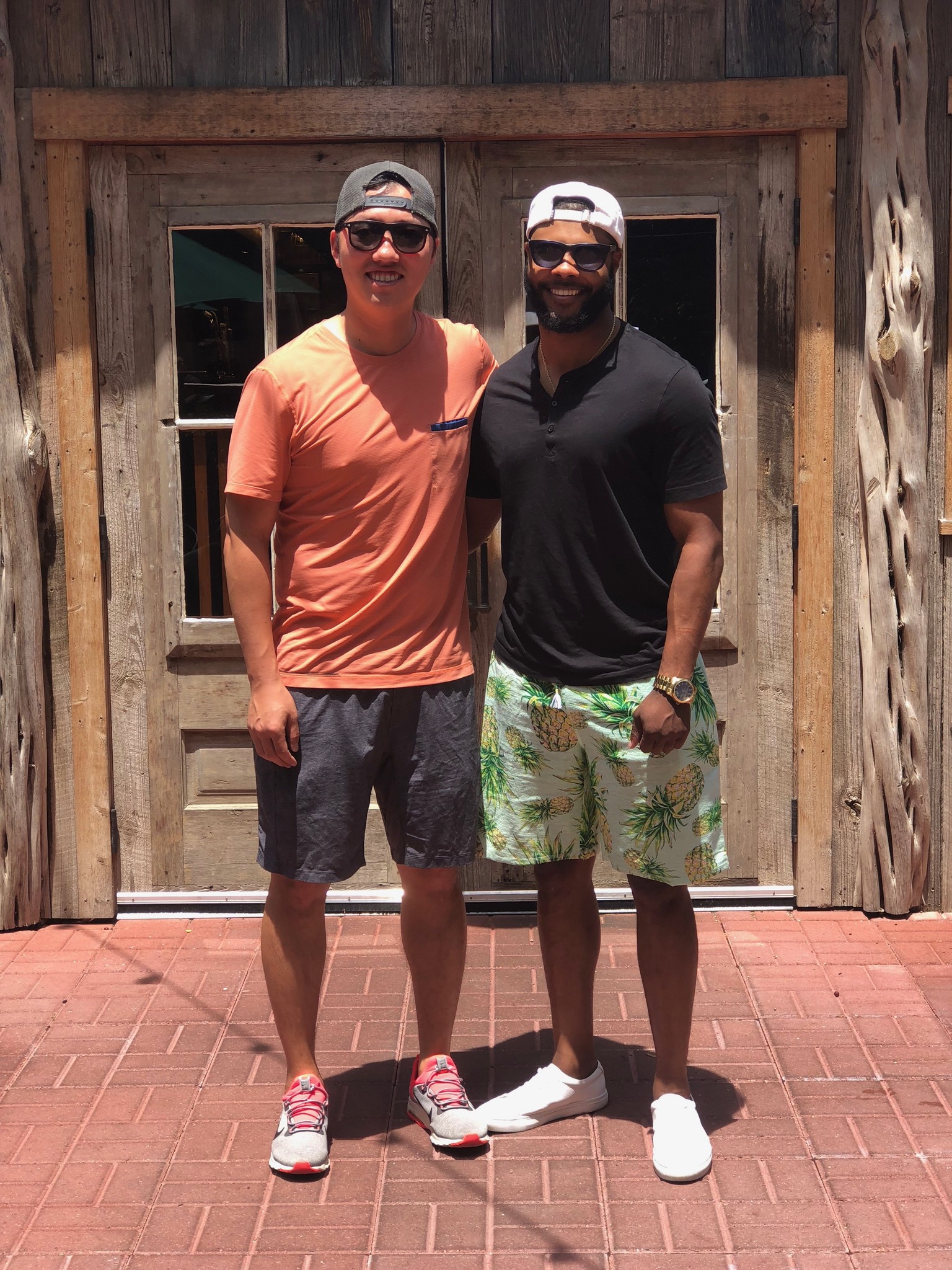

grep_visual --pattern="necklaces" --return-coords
[536,316,616,393]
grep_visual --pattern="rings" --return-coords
[660,750,666,757]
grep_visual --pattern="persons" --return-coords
[221,157,495,1176]
[462,177,727,1183]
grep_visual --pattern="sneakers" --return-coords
[269,1075,330,1174]
[478,1060,608,1132]
[650,1092,713,1183]
[407,1053,490,1148]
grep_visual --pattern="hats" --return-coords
[526,181,625,248]
[334,161,437,233]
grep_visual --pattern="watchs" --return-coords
[653,671,696,706]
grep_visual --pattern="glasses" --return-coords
[528,239,616,271]
[340,220,434,254]
